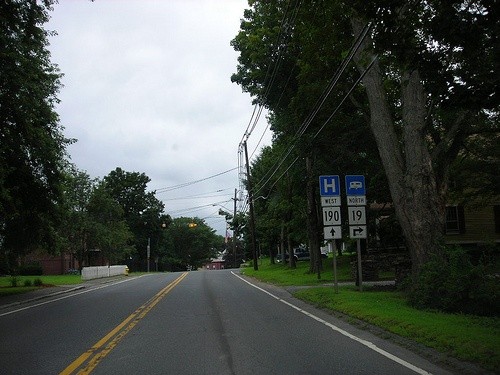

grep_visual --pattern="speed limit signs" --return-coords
[323,207,341,226]
[348,207,366,225]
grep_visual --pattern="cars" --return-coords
[277,248,330,263]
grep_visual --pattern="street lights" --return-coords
[213,203,237,268]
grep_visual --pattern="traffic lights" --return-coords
[189,223,197,228]
[161,223,168,229]
